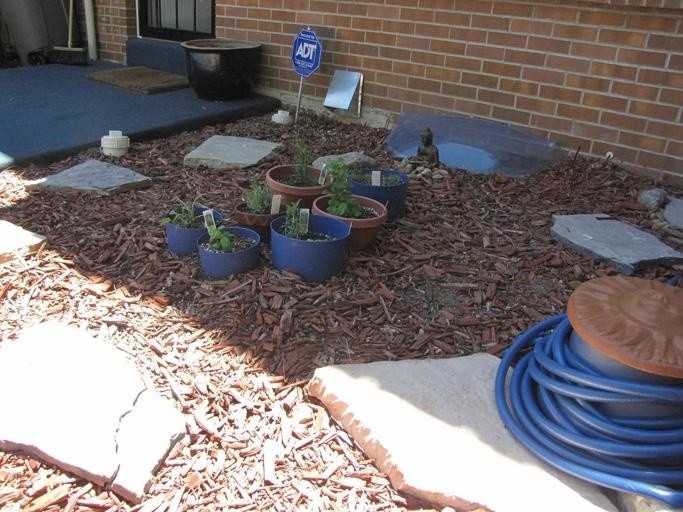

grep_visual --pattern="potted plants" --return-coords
[162,134,411,283]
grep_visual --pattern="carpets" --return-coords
[85,64,192,95]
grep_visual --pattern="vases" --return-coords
[182,36,262,100]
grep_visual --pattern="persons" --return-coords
[411,128,439,169]
